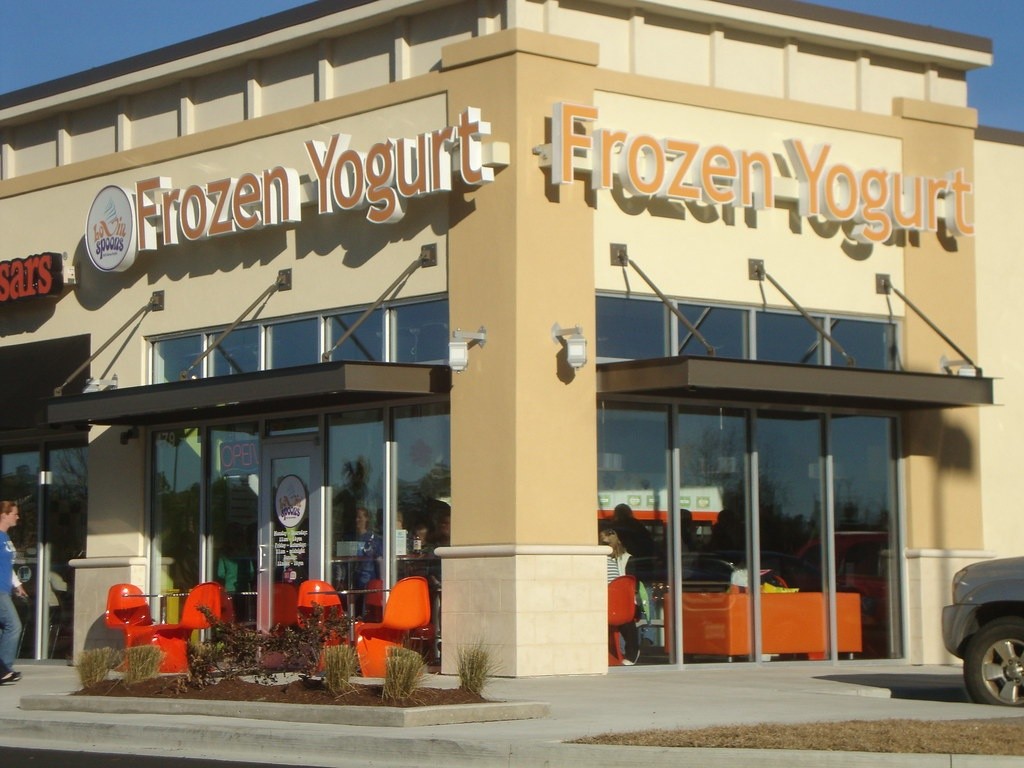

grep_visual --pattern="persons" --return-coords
[333,509,451,637]
[613,504,741,593]
[167,522,257,626]
[600,530,640,666]
[0,501,29,685]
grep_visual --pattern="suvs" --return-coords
[791,530,889,643]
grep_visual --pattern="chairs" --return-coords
[146,581,227,675]
[356,576,432,677]
[296,580,349,675]
[607,576,638,667]
[16,604,62,659]
[106,583,154,673]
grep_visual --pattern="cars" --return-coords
[616,554,796,666]
[942,556,1024,709]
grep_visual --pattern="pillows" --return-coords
[762,583,800,593]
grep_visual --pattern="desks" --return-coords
[121,593,190,624]
[307,588,391,651]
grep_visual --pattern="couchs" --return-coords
[663,591,862,663]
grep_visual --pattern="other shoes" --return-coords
[0,671,22,683]
[621,649,641,666]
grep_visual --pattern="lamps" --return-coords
[82,373,118,394]
[940,354,976,377]
[552,322,587,370]
[449,326,486,371]
